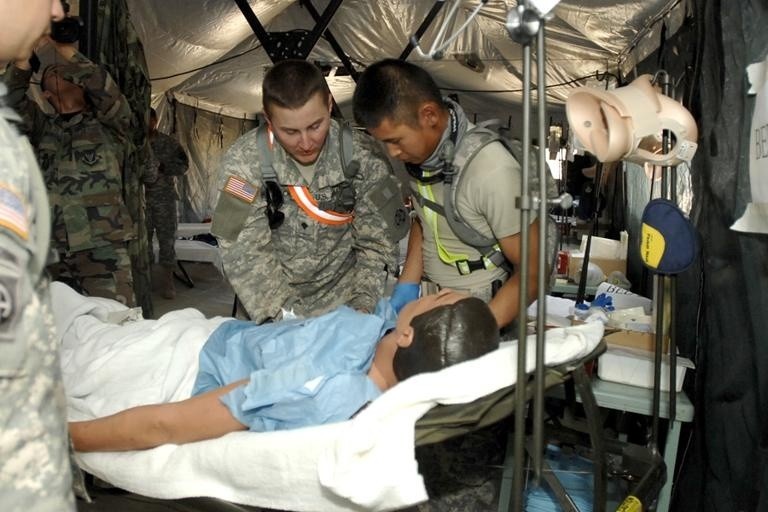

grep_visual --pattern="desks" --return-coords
[575,377,694,512]
[550,279,598,295]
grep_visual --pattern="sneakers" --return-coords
[161,277,175,299]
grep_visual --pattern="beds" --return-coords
[153,240,237,318]
[45,280,608,512]
[177,223,212,239]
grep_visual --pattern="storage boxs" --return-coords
[598,342,695,392]
[568,250,626,281]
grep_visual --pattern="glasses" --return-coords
[266,180,284,230]
[400,164,447,185]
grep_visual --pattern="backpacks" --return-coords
[442,126,560,295]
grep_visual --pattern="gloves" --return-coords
[388,282,421,312]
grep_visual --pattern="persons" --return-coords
[5,37,140,311]
[0,1,79,510]
[211,59,411,323]
[352,58,549,342]
[140,108,189,299]
[50,220,500,453]
